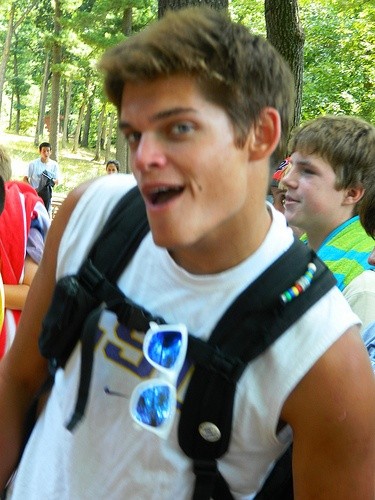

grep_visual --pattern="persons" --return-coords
[28,142,60,220]
[270,115,375,367]
[0,145,52,362]
[105,160,120,176]
[0,7,374,500]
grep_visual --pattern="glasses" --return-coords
[129,323,189,441]
